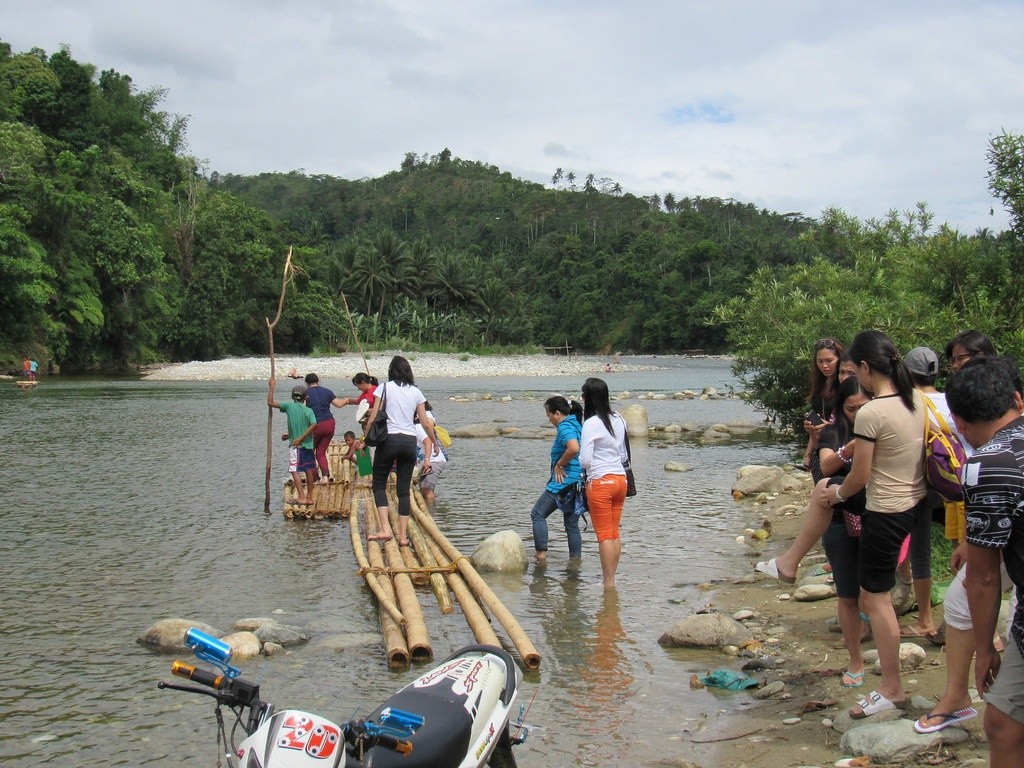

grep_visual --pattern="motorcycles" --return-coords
[155,624,540,768]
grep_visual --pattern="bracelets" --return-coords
[805,454,811,459]
[557,463,565,468]
[836,487,846,502]
[838,448,851,463]
[842,446,847,458]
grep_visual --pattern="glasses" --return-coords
[949,352,971,365]
[814,340,835,348]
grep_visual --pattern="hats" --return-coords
[904,347,939,375]
[291,386,309,399]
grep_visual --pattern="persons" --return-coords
[348,373,377,409]
[268,378,317,505]
[23,356,38,381]
[943,361,1024,768]
[413,401,447,505]
[605,364,611,372]
[358,355,439,545]
[531,396,583,559]
[288,368,297,379]
[579,377,628,586]
[304,373,349,484]
[753,330,1020,733]
[341,431,361,472]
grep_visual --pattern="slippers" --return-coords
[898,626,938,638]
[417,465,432,483]
[755,559,796,585]
[843,668,865,688]
[832,630,874,649]
[926,623,947,646]
[914,706,977,732]
[848,690,908,719]
[829,623,843,633]
[895,564,914,584]
[970,637,1004,660]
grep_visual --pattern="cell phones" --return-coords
[806,410,824,427]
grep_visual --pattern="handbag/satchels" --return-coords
[434,426,451,448]
[365,382,388,447]
[556,482,588,515]
[621,460,637,497]
[916,388,967,504]
[844,509,861,537]
